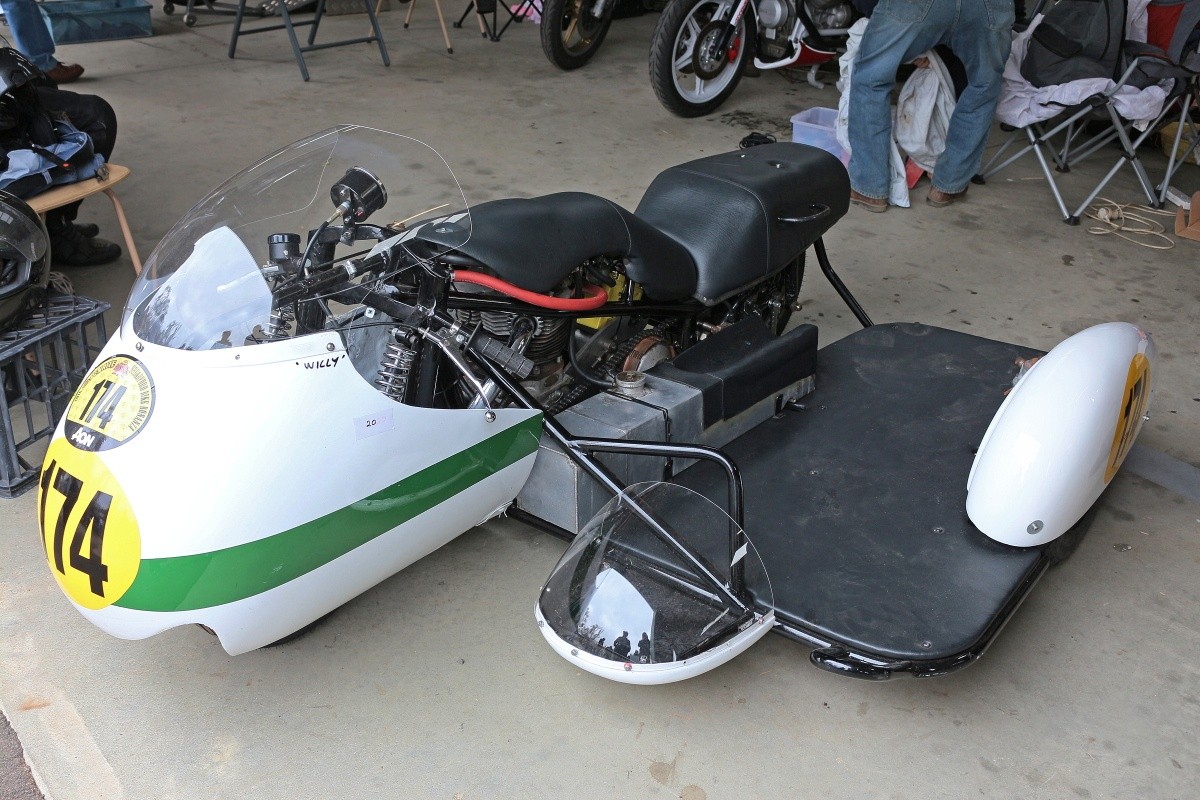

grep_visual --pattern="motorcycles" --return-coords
[541,0,879,119]
[34,121,1156,687]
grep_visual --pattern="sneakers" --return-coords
[48,222,121,267]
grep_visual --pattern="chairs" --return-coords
[26,163,142,277]
[970,1,1200,225]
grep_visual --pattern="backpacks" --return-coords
[1020,0,1129,88]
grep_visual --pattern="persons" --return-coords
[0,0,121,266]
[848,0,1017,213]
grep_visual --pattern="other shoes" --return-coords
[850,189,888,213]
[927,184,969,208]
[47,62,84,84]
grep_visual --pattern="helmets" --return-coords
[0,190,51,332]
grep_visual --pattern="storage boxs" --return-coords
[38,0,153,45]
[0,288,112,499]
[790,107,851,172]
